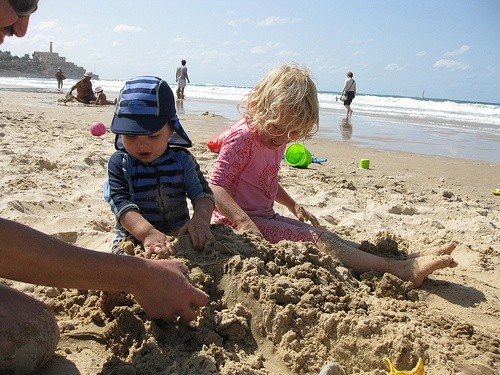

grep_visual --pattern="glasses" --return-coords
[8,0,39,17]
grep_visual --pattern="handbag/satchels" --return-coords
[340,90,351,102]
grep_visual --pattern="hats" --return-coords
[110,75,195,150]
[83,70,93,78]
[94,86,104,93]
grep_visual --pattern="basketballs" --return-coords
[90,121,105,136]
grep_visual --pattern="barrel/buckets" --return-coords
[284,144,311,168]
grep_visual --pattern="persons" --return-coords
[342,71,356,116]
[54,67,66,88]
[176,60,190,98]
[98,76,214,312]
[69,71,117,105]
[206,62,460,289]
[0,2,206,374]
[92,88,107,105]
